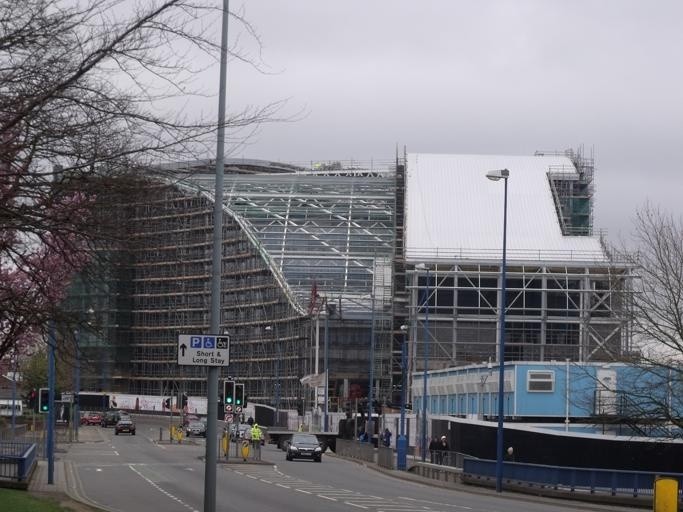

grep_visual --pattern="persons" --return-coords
[355,426,369,442]
[252,423,262,448]
[432,436,440,463]
[438,435,448,464]
[382,428,391,447]
[429,435,434,463]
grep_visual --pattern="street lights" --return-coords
[45,307,95,486]
[413,262,431,463]
[264,325,282,407]
[485,168,509,491]
[397,324,407,469]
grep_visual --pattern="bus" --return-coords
[62,392,109,417]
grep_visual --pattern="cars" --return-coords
[78,408,136,436]
[184,413,264,446]
[284,431,322,463]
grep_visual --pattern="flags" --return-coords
[299,370,326,387]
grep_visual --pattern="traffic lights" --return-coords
[30,388,36,408]
[176,392,187,409]
[37,387,51,414]
[233,383,244,407]
[223,380,234,406]
